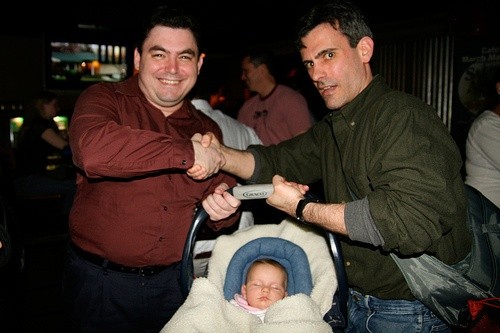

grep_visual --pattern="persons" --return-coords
[67,19,240,321]
[187,9,475,333]
[237,42,316,147]
[159,220,338,333]
[458,36,500,295]
[15,83,76,198]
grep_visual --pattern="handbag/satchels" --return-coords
[392,249,498,330]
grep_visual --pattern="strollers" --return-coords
[177,183,349,333]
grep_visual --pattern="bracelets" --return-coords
[296,195,318,227]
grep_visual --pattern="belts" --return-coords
[71,244,180,278]
